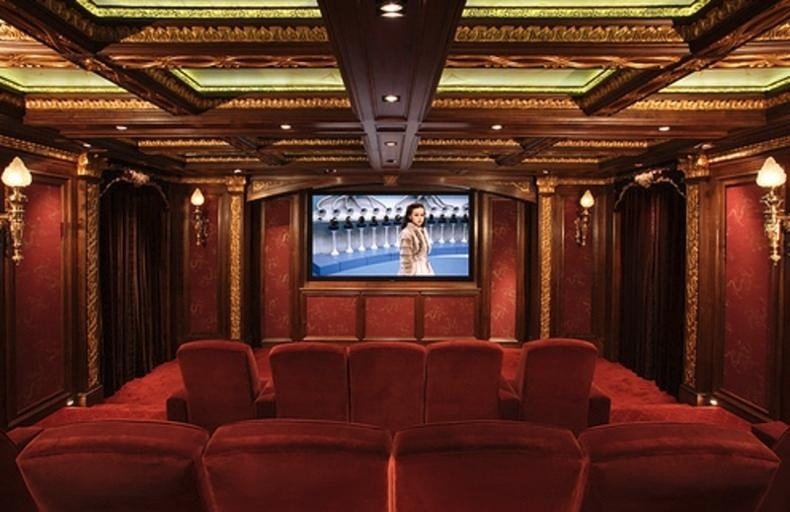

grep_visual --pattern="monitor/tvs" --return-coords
[307,193,472,282]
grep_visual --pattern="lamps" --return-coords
[576,189,596,249]
[1,156,32,267]
[755,157,790,267]
[189,188,209,248]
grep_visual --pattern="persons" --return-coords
[397,203,435,277]
